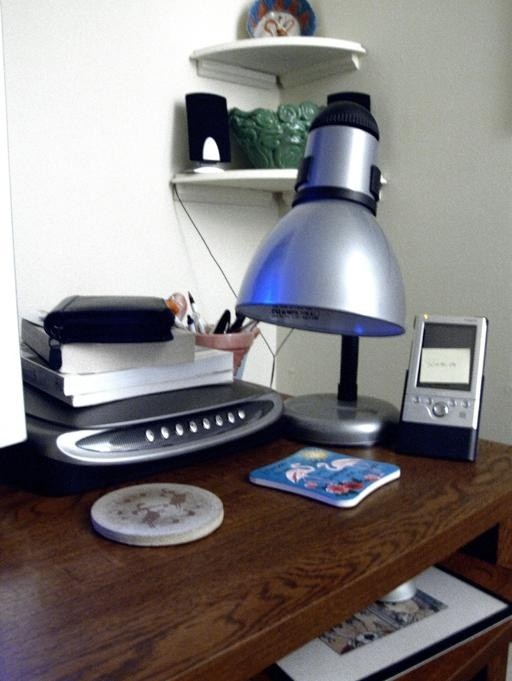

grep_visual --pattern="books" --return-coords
[20,316,198,369]
[23,370,235,410]
[20,349,233,398]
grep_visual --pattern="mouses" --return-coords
[376,578,417,602]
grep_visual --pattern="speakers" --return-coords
[186,92,232,165]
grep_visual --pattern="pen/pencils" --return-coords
[213,309,230,334]
[186,314,196,334]
[188,291,205,335]
[229,315,245,332]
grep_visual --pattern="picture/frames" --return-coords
[274,562,511,680]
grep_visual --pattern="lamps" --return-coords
[236,92,405,448]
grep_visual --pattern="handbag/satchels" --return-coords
[43,295,176,370]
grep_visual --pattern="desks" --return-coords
[1,394,512,681]
